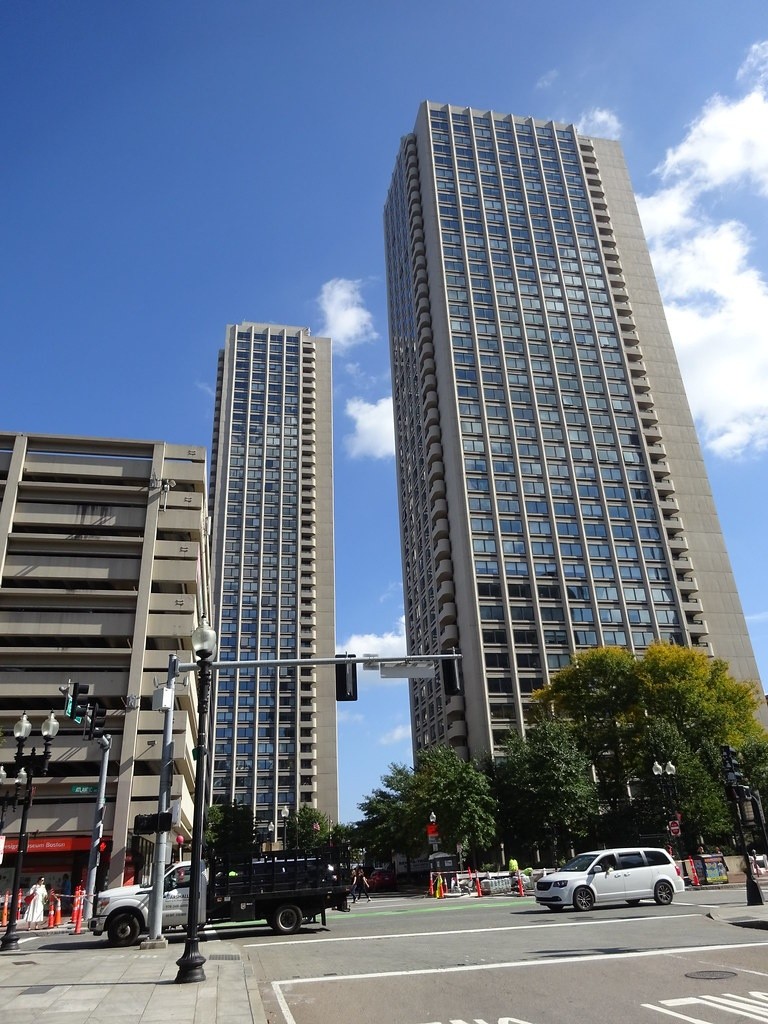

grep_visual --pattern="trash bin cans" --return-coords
[694,853,728,884]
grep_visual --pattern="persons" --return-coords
[511,879,526,892]
[602,857,614,871]
[350,870,358,903]
[667,845,672,855]
[715,847,730,884]
[697,846,706,854]
[61,874,71,913]
[25,876,49,930]
[356,869,372,903]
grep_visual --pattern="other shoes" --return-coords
[367,897,371,903]
[352,898,358,903]
[26,927,40,931]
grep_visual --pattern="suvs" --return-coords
[366,870,398,892]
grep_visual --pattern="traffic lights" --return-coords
[99,839,113,856]
[71,683,89,720]
[740,785,752,801]
[154,811,171,833]
[723,749,743,783]
[88,703,106,741]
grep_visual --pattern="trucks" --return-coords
[88,846,354,947]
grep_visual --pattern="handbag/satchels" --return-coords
[24,893,35,906]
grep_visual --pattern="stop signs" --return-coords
[667,821,680,836]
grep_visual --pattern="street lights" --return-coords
[0,710,61,952]
[268,821,274,850]
[282,805,289,851]
[653,759,680,856]
[174,614,217,983]
[0,765,27,834]
[430,811,437,852]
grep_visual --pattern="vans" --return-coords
[751,856,768,875]
[533,847,685,913]
